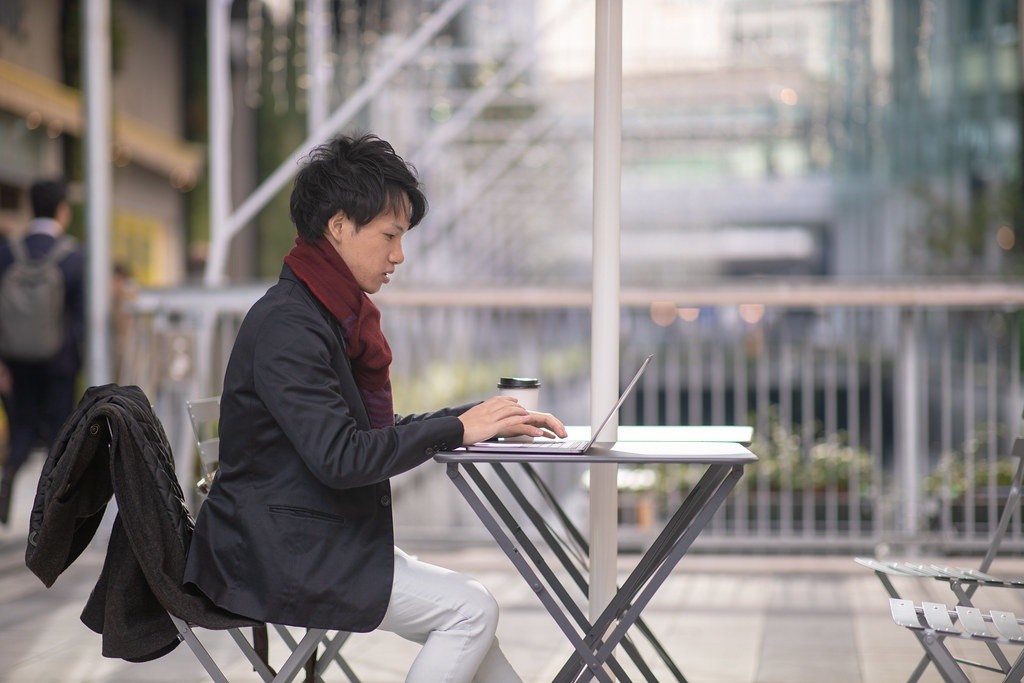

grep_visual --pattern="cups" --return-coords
[497,377,541,442]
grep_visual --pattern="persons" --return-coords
[0,179,88,521]
[181,134,568,683]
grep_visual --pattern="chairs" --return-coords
[888,598,1024,683]
[854,410,1024,683]
[104,396,362,683]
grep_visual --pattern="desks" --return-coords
[432,424,759,683]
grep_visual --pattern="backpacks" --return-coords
[0,233,75,361]
